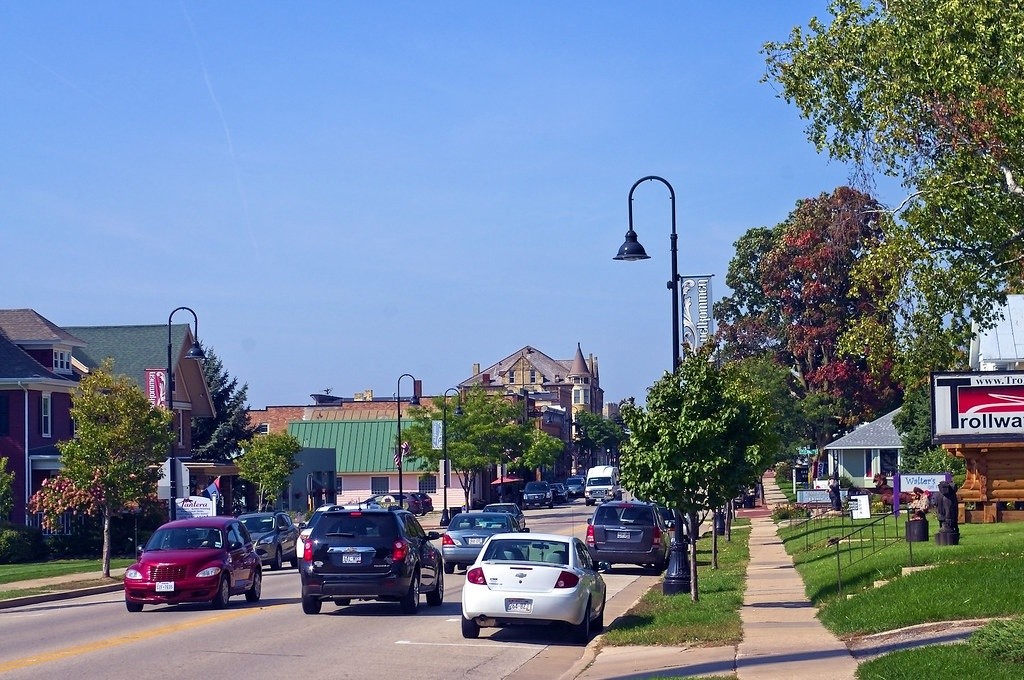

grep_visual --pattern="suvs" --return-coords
[236,511,299,571]
[301,506,447,614]
[586,499,671,571]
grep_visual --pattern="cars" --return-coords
[442,513,530,575]
[549,475,588,504]
[479,503,526,532]
[124,517,263,612]
[296,504,380,574]
[357,492,435,517]
[519,481,556,511]
[458,534,612,646]
[617,500,691,540]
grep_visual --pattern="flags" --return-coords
[202,476,220,504]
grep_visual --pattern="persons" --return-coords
[494,483,524,503]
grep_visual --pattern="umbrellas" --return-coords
[491,474,524,484]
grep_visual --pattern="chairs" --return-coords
[460,523,471,529]
[170,531,187,548]
[547,550,569,565]
[490,524,503,529]
[499,548,524,561]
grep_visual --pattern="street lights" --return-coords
[167,307,206,520]
[397,373,422,507]
[612,175,694,593]
[439,387,465,526]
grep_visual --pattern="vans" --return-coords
[580,465,622,506]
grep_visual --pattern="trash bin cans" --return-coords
[450,507,462,521]
[744,495,755,508]
